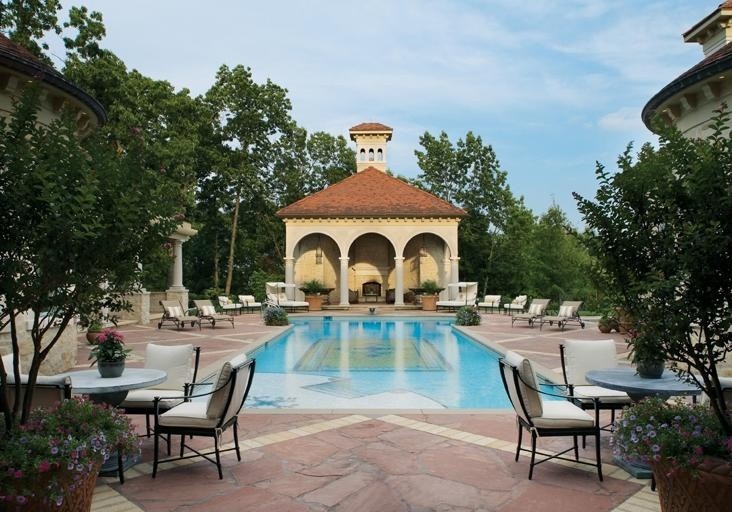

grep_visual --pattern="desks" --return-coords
[586,367,731,481]
[47,365,170,479]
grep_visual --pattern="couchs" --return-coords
[386,288,416,304]
[348,289,358,303]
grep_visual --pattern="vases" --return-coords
[1,460,104,512]
[646,453,732,512]
[611,306,632,334]
[99,358,125,378]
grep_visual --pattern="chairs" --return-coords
[499,346,604,483]
[150,353,257,478]
[121,344,200,456]
[436,281,585,330]
[0,351,72,410]
[153,281,311,330]
[558,335,636,448]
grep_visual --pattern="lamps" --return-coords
[316,235,323,265]
[418,234,427,256]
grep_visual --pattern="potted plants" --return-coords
[627,298,669,376]
[597,312,610,334]
[417,279,441,310]
[299,277,322,310]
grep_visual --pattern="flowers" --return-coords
[87,329,133,367]
[0,76,195,512]
[572,101,732,512]
